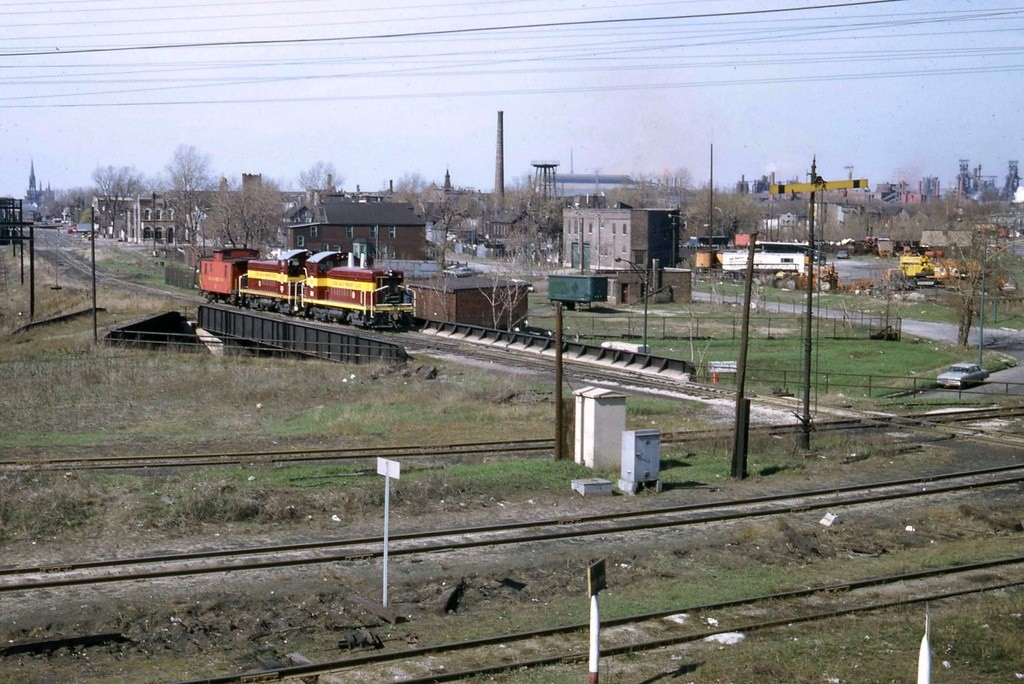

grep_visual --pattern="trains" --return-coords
[196,247,416,331]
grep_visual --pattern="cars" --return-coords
[838,250,849,260]
[937,363,989,390]
